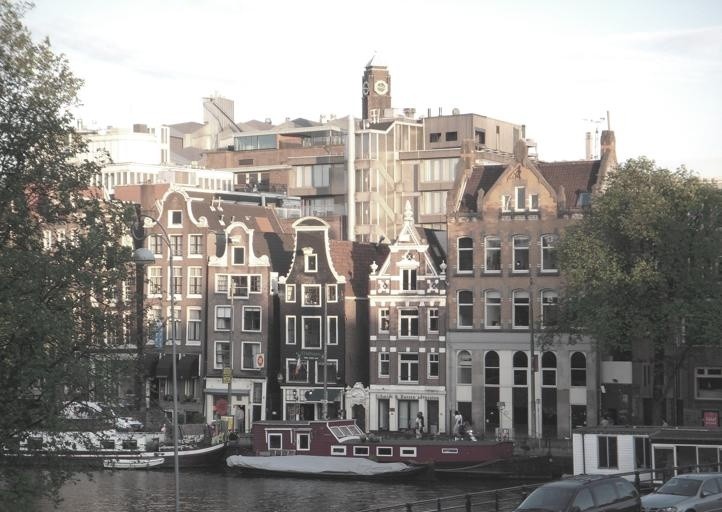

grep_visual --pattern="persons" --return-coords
[600,416,609,426]
[452,410,461,434]
[236,406,244,431]
[661,417,669,426]
[414,412,423,439]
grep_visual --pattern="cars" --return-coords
[515,473,722,512]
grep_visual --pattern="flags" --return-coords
[295,358,302,375]
